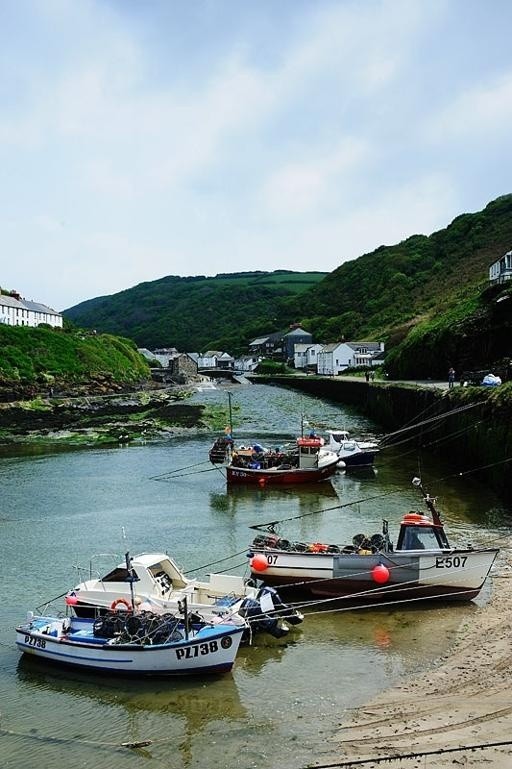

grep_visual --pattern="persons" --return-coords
[447,367,456,388]
[365,369,369,381]
[370,370,375,381]
[460,375,465,386]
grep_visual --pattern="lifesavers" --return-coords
[111,598,131,615]
[404,514,422,519]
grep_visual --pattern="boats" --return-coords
[209,392,380,483]
[247,486,501,601]
